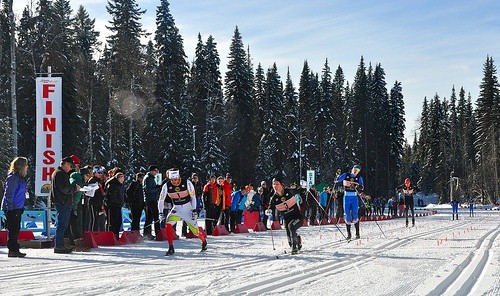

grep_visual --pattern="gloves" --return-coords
[193,212,199,220]
[159,214,166,224]
[350,183,358,188]
[265,209,272,217]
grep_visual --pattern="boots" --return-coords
[346,224,351,239]
[74,239,90,252]
[354,220,360,239]
[64,237,73,250]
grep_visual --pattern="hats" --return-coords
[80,168,90,174]
[168,168,180,179]
[405,179,411,184]
[70,154,80,164]
[149,165,157,171]
[61,157,76,167]
[354,165,361,173]
[272,177,282,185]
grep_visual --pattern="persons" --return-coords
[88,165,106,231]
[450,200,461,220]
[142,165,161,239]
[202,175,217,234]
[250,187,263,222]
[52,157,79,253]
[319,186,327,221]
[219,173,232,234]
[397,178,418,227]
[64,155,404,252]
[308,185,318,225]
[108,173,126,238]
[266,178,307,255]
[1,157,28,257]
[230,187,247,232]
[127,173,145,236]
[337,165,364,240]
[158,168,208,256]
[467,199,475,217]
[418,198,424,205]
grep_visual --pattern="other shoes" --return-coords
[202,241,208,251]
[291,248,299,254]
[165,245,175,256]
[54,248,72,254]
[297,236,302,250]
[8,247,26,257]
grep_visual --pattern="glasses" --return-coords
[68,164,72,168]
